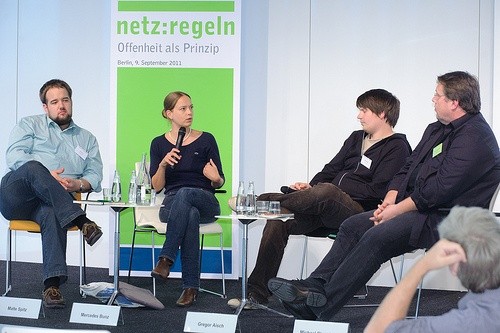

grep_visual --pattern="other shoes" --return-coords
[228,194,256,213]
[227,292,267,310]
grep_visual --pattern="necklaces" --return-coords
[168,127,191,142]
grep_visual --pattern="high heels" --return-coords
[151,258,172,280]
[175,288,197,306]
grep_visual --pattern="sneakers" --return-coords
[81,221,103,246]
[41,286,65,307]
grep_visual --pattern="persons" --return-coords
[0,79,103,308]
[149,91,225,305]
[362,204,500,333]
[268,70,500,322]
[227,89,412,310]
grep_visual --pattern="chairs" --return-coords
[0,191,87,301]
[127,203,228,298]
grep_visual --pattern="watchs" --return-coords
[78,180,84,192]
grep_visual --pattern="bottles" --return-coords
[136,152,151,205]
[245,181,256,216]
[236,181,246,215]
[128,171,137,203]
[111,170,122,202]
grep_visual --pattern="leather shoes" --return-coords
[279,297,315,321]
[268,276,328,308]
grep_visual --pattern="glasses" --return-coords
[434,90,446,98]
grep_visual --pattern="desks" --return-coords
[214,214,293,319]
[72,201,165,309]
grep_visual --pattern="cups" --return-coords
[257,200,269,215]
[269,201,280,215]
[151,189,156,204]
[103,188,112,199]
